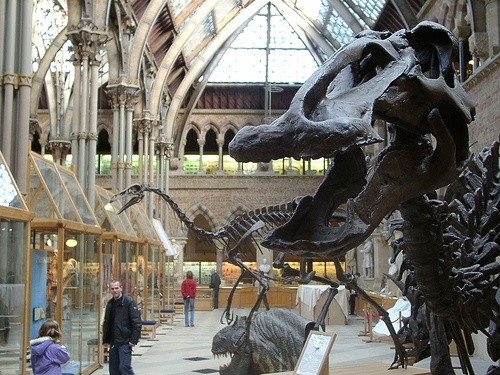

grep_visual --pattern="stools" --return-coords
[174,301,185,315]
[140,320,158,341]
[87,338,99,362]
[159,310,176,324]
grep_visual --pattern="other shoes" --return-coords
[185,323,194,327]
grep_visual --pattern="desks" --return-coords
[298,285,347,325]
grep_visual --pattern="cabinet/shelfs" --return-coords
[174,285,300,311]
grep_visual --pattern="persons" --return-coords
[102,279,142,375]
[360,238,374,278]
[255,271,270,312]
[208,267,221,309]
[348,272,360,316]
[181,270,196,327]
[29,319,70,375]
